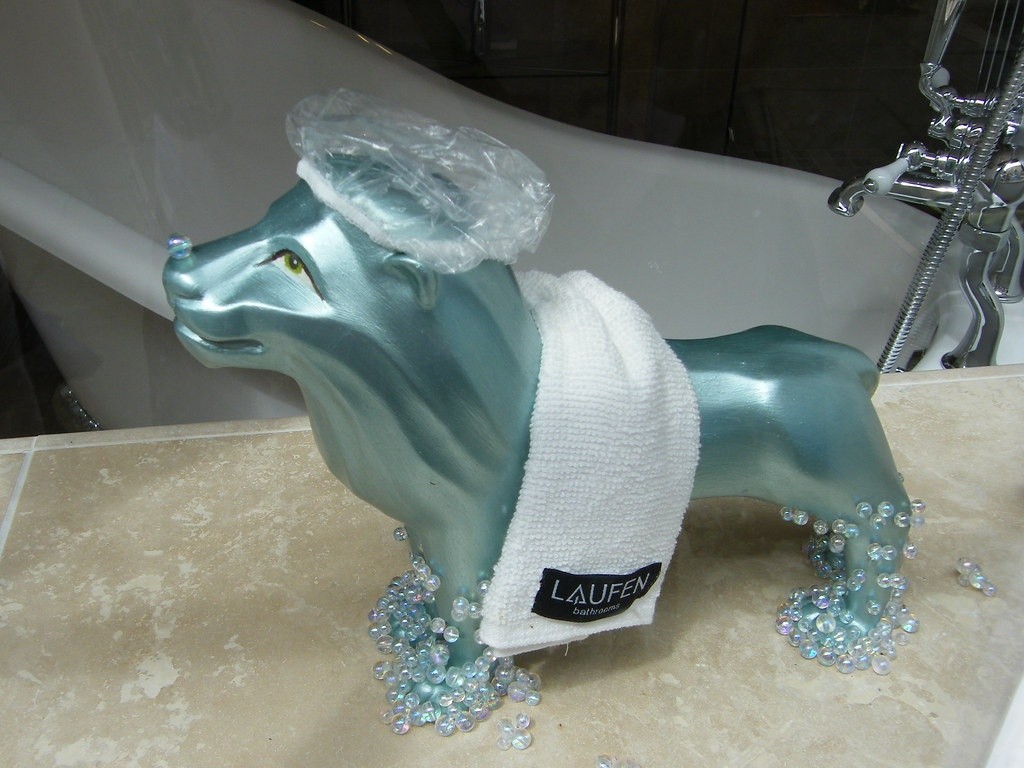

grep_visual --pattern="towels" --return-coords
[475,266,704,660]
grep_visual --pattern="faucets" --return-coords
[825,176,1024,305]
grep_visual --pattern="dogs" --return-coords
[159,150,911,722]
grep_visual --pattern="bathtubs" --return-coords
[0,0,1023,431]
